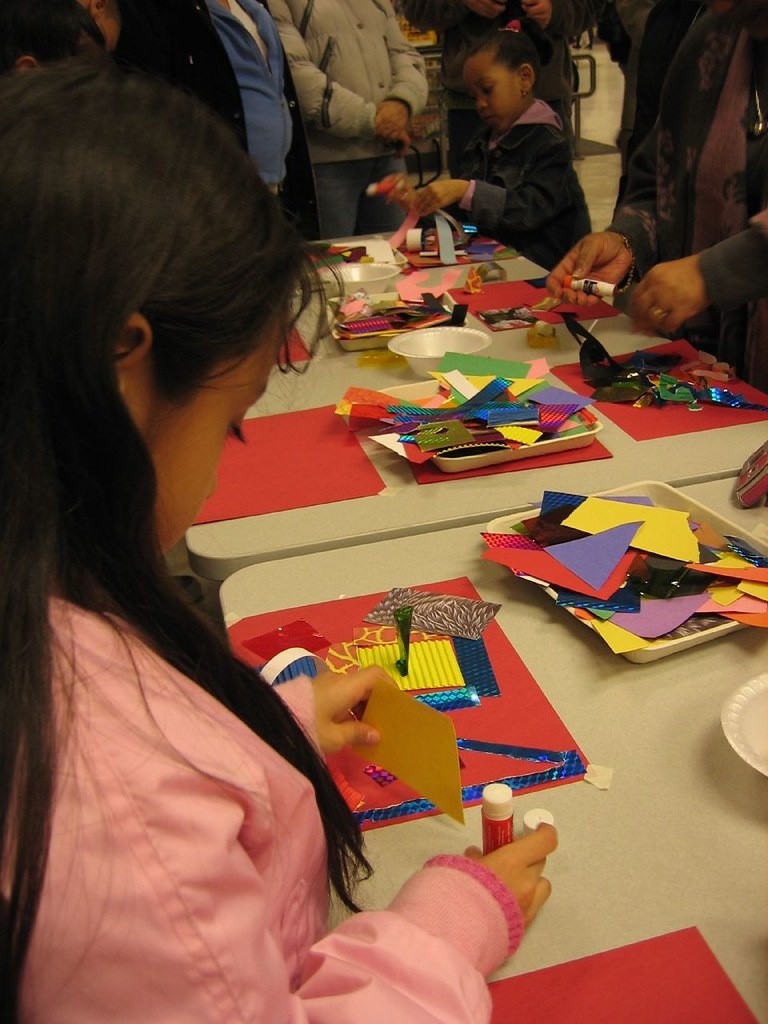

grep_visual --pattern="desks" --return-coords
[217,470,768,1024]
[182,227,768,582]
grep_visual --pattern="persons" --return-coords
[80,1,322,244]
[1,1,107,87]
[0,62,558,1023]
[546,0,768,396]
[402,0,605,181]
[266,0,430,239]
[365,16,591,289]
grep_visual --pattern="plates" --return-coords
[721,671,768,779]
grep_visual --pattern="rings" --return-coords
[652,304,667,319]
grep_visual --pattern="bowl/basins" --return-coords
[388,327,492,378]
[320,263,402,296]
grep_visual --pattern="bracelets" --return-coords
[617,232,636,295]
